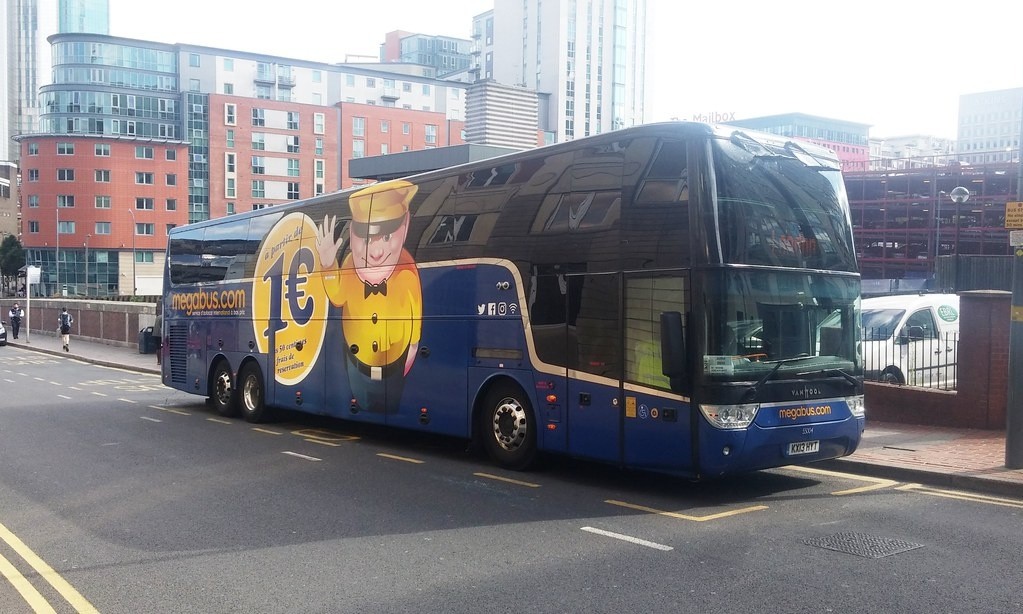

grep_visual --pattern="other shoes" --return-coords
[17,337,19,339]
[63,345,65,351]
[14,337,16,339]
[66,345,69,352]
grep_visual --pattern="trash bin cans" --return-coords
[139,326,156,354]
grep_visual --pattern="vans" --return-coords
[815,291,961,390]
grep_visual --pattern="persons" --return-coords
[152,314,162,365]
[57,306,74,353]
[9,303,25,339]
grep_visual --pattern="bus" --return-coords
[159,117,867,482]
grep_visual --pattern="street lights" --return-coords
[951,187,971,295]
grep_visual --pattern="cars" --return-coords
[733,318,769,361]
